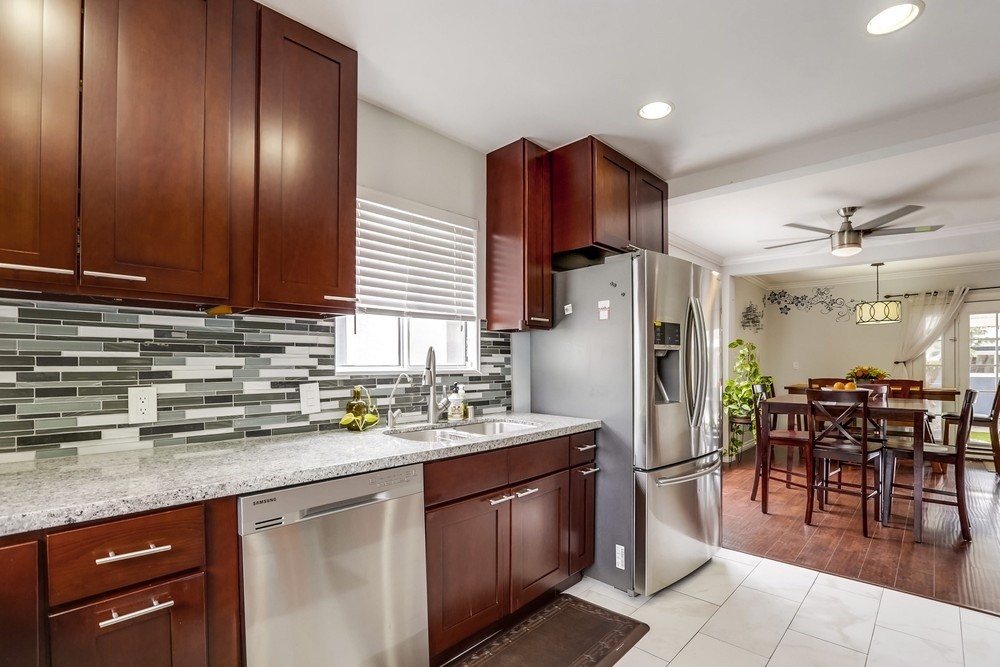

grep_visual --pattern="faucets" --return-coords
[386,373,411,428]
[420,346,451,422]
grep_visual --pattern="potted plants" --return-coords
[722,339,773,458]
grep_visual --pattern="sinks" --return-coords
[444,418,543,437]
[383,424,482,444]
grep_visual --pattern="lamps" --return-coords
[855,263,903,325]
[830,232,864,257]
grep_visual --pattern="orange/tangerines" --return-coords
[833,382,856,390]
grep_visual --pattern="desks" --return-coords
[783,382,961,459]
[758,393,929,543]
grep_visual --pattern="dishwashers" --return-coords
[237,463,430,667]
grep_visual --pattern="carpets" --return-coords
[442,593,651,667]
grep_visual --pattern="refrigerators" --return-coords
[532,249,720,598]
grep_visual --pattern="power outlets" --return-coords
[128,386,158,424]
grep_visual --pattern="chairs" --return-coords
[941,379,1000,477]
[750,377,978,542]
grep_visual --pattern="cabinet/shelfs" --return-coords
[550,135,670,269]
[0,0,358,320]
[485,138,553,332]
[0,498,245,667]
[424,436,569,667]
[569,430,600,575]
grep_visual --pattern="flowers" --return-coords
[846,366,893,383]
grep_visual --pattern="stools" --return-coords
[728,416,752,466]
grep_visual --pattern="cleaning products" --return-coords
[448,381,464,421]
[457,385,467,419]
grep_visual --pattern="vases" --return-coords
[853,377,872,384]
[345,388,366,431]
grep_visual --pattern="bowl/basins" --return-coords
[821,387,874,396]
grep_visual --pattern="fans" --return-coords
[765,204,945,250]
